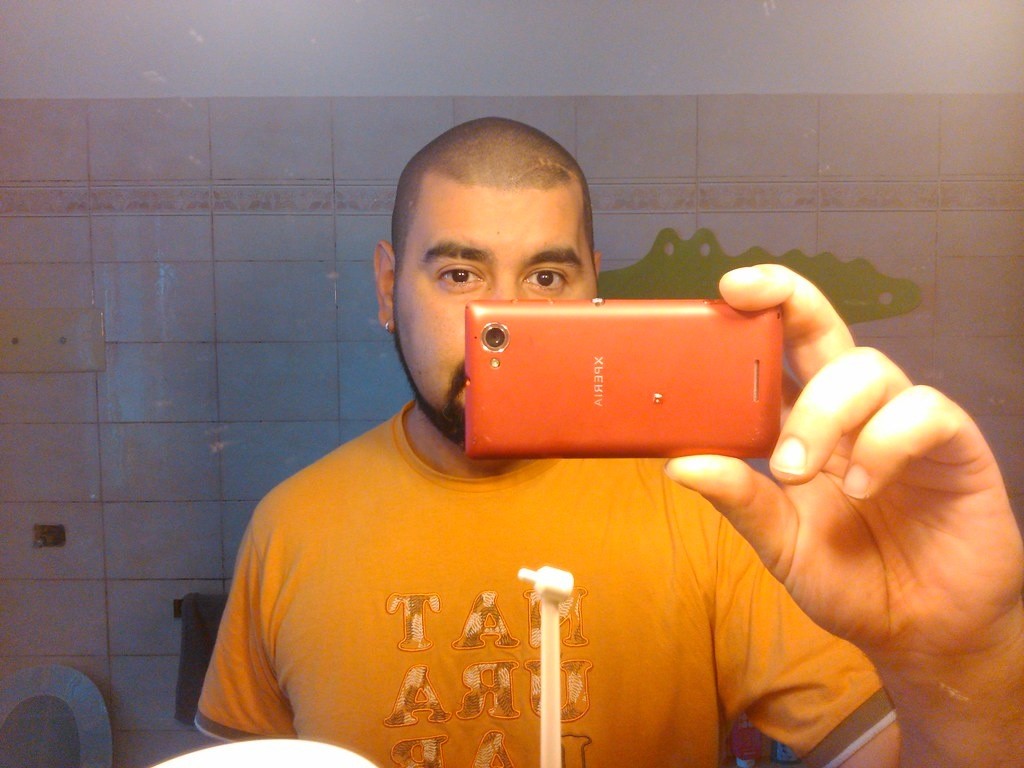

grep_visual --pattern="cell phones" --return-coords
[466,299,784,460]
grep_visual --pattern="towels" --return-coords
[174,591,229,724]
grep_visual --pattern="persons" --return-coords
[194,115,1024,768]
